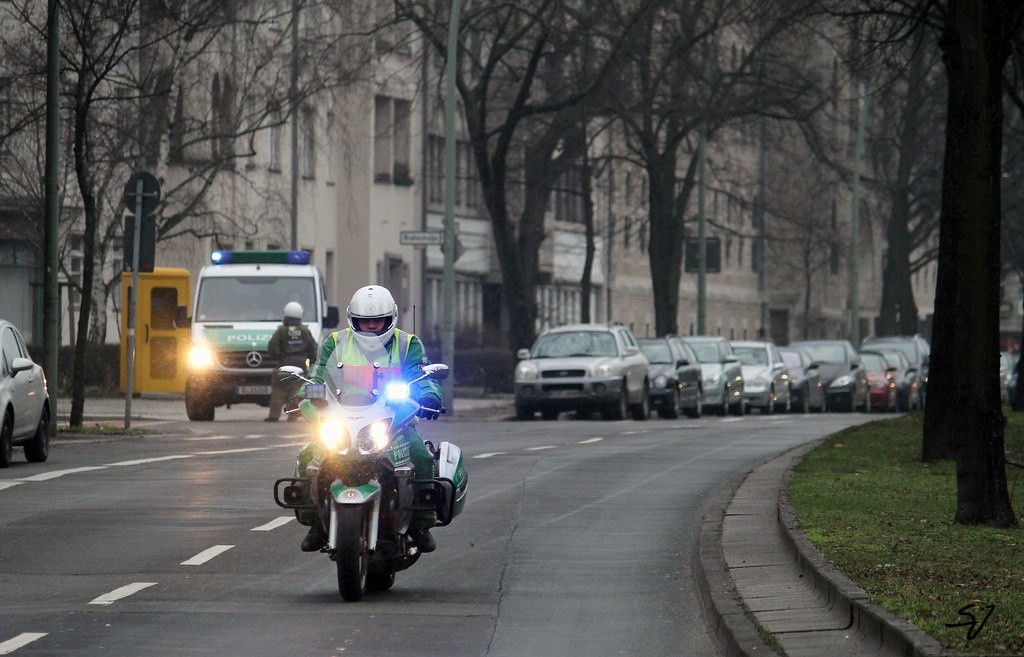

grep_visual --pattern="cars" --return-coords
[857,351,899,413]
[756,347,827,414]
[875,348,923,413]
[729,341,791,414]
[0,318,52,469]
[999,351,1018,401]
[681,335,746,417]
[789,340,871,413]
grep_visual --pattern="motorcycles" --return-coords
[273,366,468,602]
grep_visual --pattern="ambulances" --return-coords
[173,250,339,422]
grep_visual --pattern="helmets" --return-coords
[346,285,398,351]
[284,302,303,319]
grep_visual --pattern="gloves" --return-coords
[284,397,303,415]
[416,397,442,420]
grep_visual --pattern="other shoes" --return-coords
[287,413,302,422]
[265,418,278,421]
[409,528,436,553]
[301,527,325,551]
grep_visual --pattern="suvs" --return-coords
[634,333,704,419]
[513,320,651,421]
[858,333,931,409]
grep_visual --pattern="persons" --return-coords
[262,299,319,421]
[286,283,443,556]
